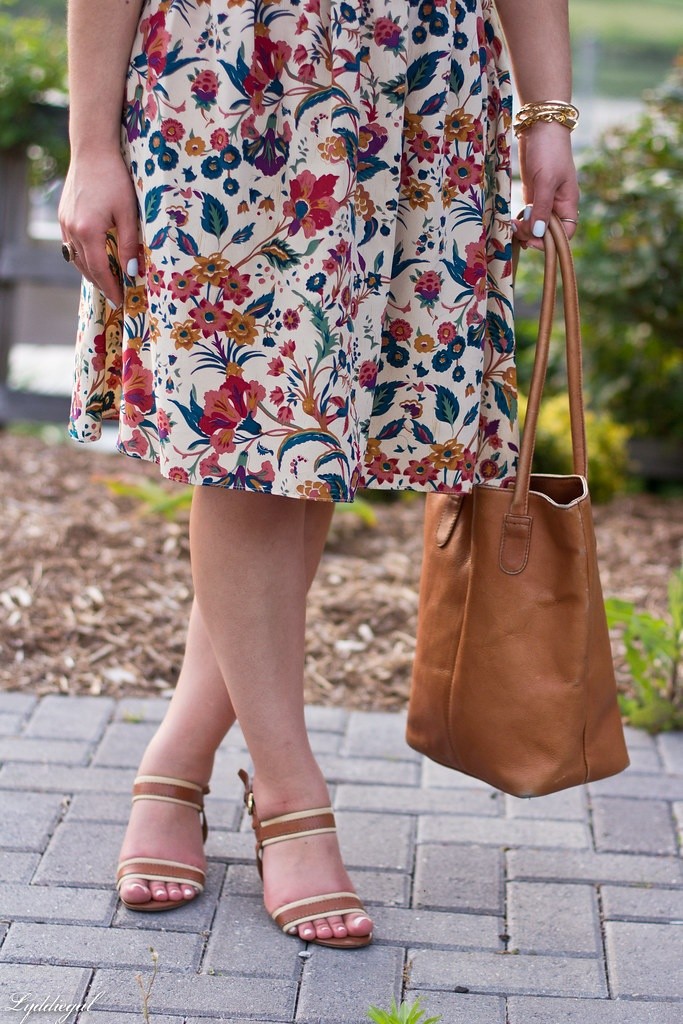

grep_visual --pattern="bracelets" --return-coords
[514,101,579,136]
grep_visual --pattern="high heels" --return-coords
[234,768,374,952]
[113,774,214,912]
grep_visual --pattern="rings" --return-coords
[562,219,578,225]
[61,242,79,261]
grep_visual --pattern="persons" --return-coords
[59,0,579,948]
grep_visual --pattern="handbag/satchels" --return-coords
[404,191,629,798]
